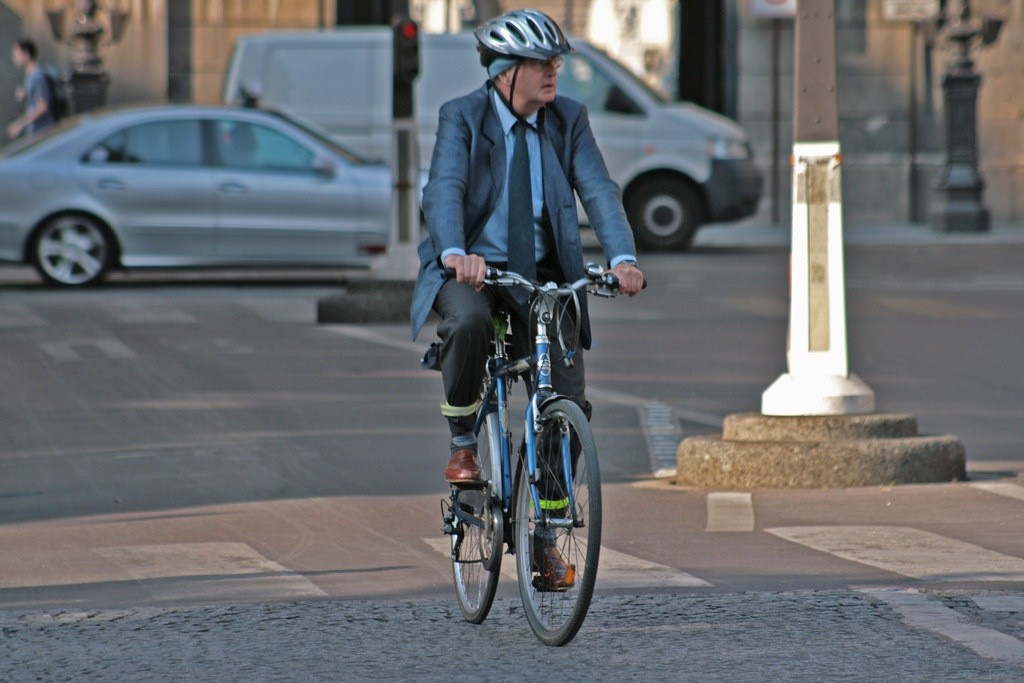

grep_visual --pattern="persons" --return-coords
[409,9,645,591]
[8,38,70,137]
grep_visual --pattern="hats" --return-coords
[489,59,518,81]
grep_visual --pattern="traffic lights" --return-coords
[392,20,421,76]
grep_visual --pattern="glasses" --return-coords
[523,56,563,69]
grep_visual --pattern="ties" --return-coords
[505,121,537,304]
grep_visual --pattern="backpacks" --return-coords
[29,72,70,121]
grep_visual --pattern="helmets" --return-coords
[473,9,571,67]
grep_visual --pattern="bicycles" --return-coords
[439,261,648,646]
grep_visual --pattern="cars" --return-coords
[0,106,429,289]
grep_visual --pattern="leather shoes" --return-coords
[444,449,481,484]
[531,546,576,590]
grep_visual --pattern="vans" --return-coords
[222,27,763,252]
